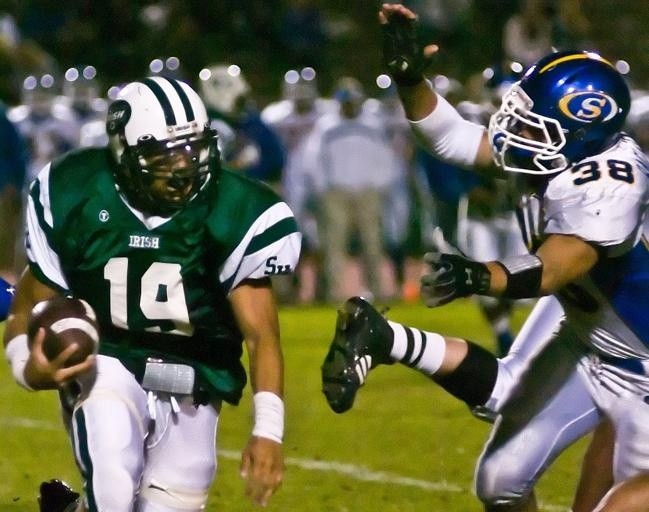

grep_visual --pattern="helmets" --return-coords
[105,77,220,206]
[487,52,631,175]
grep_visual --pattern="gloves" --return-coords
[380,17,432,86]
[419,227,490,308]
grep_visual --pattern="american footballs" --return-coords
[26,298,100,367]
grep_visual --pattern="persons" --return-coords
[373,4,649,511]
[318,91,649,414]
[4,76,304,512]
[1,0,649,366]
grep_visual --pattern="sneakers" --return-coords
[323,296,394,413]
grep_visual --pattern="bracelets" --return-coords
[6,334,42,391]
[492,253,544,297]
[251,392,288,448]
